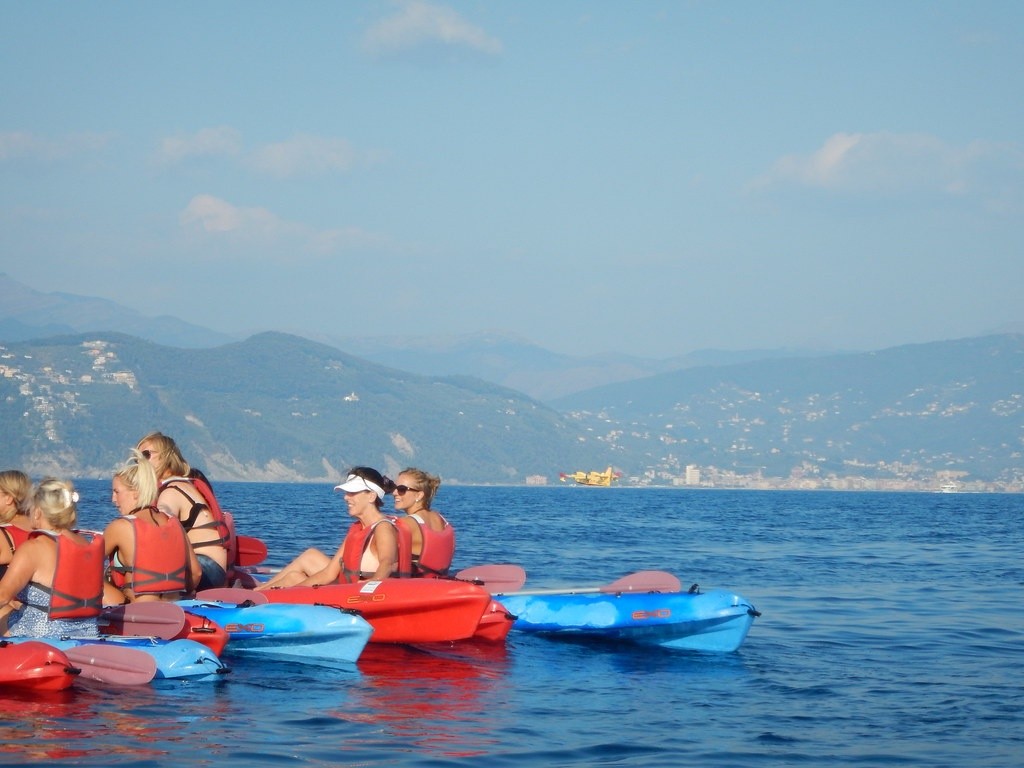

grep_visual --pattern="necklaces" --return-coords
[413,506,427,515]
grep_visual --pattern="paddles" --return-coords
[63,642,159,687]
[454,563,527,593]
[194,587,270,608]
[234,534,268,566]
[100,600,187,642]
[490,570,682,596]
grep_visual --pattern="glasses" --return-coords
[392,484,419,496]
[134,450,158,464]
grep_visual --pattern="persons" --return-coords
[0,477,105,648]
[252,465,456,594]
[291,465,400,586]
[136,430,228,594]
[0,469,36,602]
[91,456,203,605]
[186,467,238,588]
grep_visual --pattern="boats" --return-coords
[96,601,230,659]
[472,597,521,645]
[559,466,622,487]
[255,574,490,645]
[0,640,83,692]
[490,578,765,652]
[169,592,375,663]
[0,629,234,683]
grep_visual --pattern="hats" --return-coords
[334,474,385,499]
